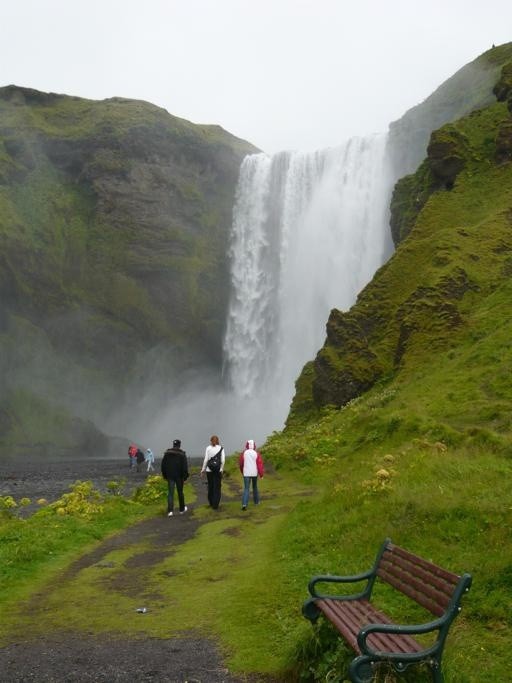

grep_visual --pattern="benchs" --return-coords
[301,537,473,682]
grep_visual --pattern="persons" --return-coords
[239,438,265,510]
[133,447,146,473]
[145,447,155,472]
[128,441,137,471]
[160,438,191,517]
[200,434,227,509]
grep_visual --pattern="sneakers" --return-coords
[168,511,173,516]
[179,505,188,513]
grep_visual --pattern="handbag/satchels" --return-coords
[207,447,223,473]
